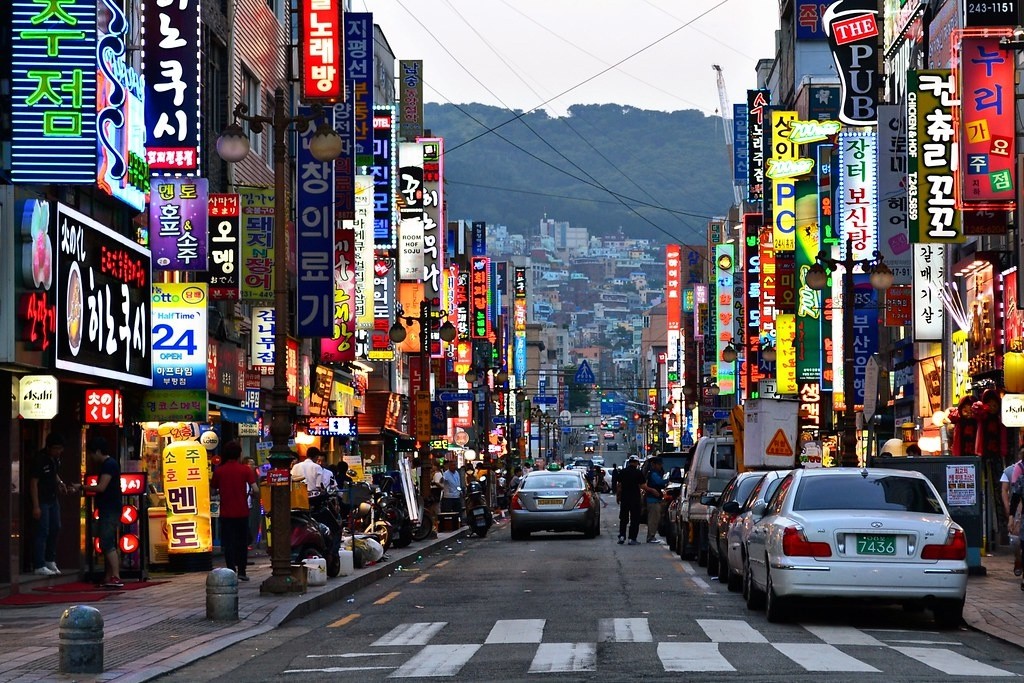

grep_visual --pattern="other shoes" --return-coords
[1013,558,1022,576]
[650,538,661,543]
[628,539,641,546]
[246,562,255,566]
[617,536,625,544]
[238,574,250,581]
[1020,578,1024,591]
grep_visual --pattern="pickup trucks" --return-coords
[670,437,737,565]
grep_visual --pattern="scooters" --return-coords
[457,476,493,538]
[266,469,431,564]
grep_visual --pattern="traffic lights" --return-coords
[585,384,650,450]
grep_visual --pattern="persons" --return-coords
[28,432,68,574]
[210,440,260,580]
[509,467,522,488]
[617,454,660,545]
[290,447,353,512]
[465,462,487,485]
[645,457,669,544]
[999,444,1024,591]
[611,463,619,496]
[67,436,124,587]
[433,459,461,531]
[523,463,533,474]
[587,465,608,508]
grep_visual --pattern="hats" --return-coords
[629,455,640,465]
[652,457,665,465]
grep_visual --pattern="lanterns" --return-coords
[1003,350,1024,393]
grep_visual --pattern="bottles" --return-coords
[336,547,353,577]
[301,556,327,586]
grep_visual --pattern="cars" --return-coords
[746,467,967,631]
[722,471,791,596]
[607,442,618,451]
[563,451,688,551]
[699,472,766,583]
[510,471,600,540]
[588,436,599,445]
[604,431,614,439]
[584,442,595,453]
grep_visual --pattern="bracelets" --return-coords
[80,485,82,490]
[57,481,63,484]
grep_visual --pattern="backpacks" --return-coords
[467,470,476,483]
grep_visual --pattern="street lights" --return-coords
[682,383,720,439]
[466,366,507,507]
[492,385,561,508]
[217,85,347,596]
[723,338,776,398]
[805,250,895,469]
[389,307,457,538]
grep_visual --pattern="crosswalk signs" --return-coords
[575,359,595,383]
